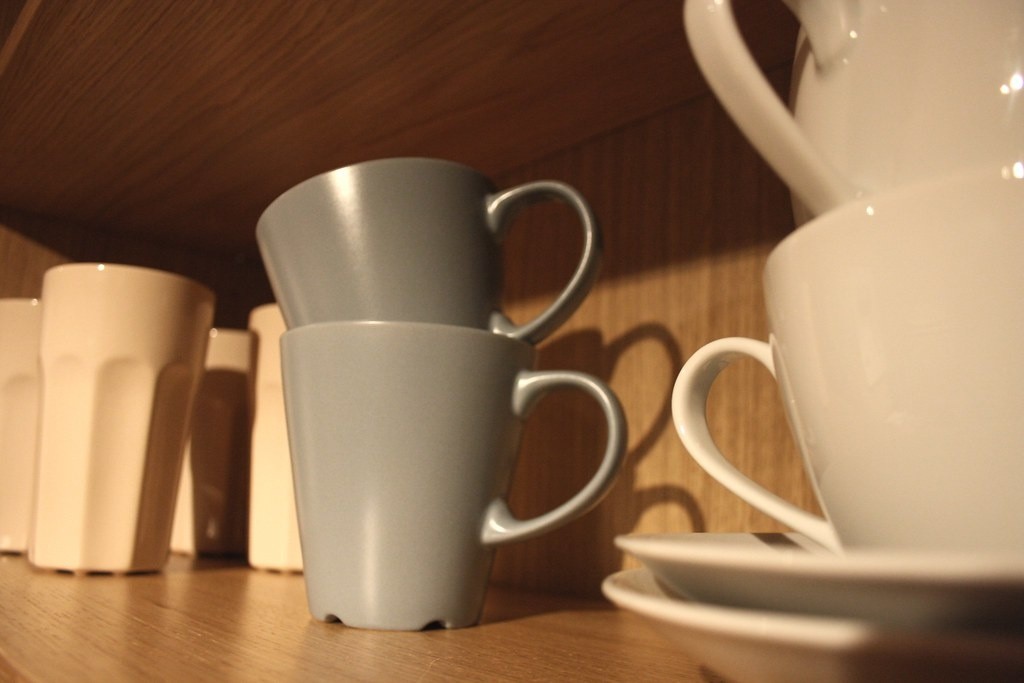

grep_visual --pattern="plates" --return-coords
[600,531,1024,683]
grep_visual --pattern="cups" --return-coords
[0,297,47,559]
[278,320,627,632]
[26,261,216,579]
[670,170,1024,554]
[253,157,603,348]
[665,0,1024,230]
[160,325,262,565]
[245,304,302,576]
